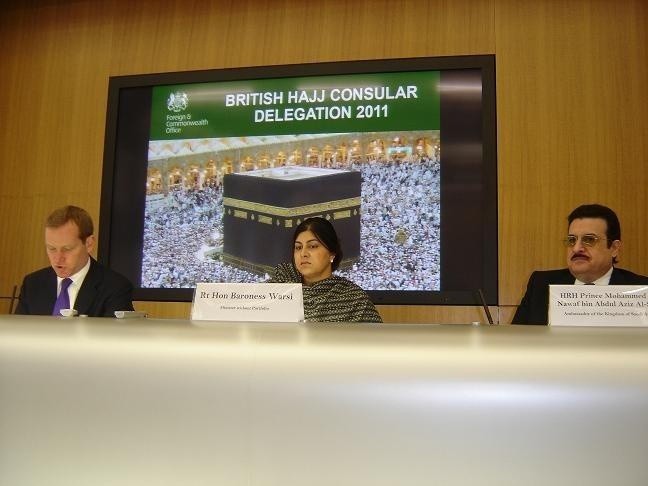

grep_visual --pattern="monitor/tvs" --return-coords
[94,52,500,307]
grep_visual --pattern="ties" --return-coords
[53,278,73,316]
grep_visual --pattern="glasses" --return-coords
[563,234,612,246]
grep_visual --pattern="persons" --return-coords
[265,217,383,323]
[15,206,135,317]
[510,204,648,325]
